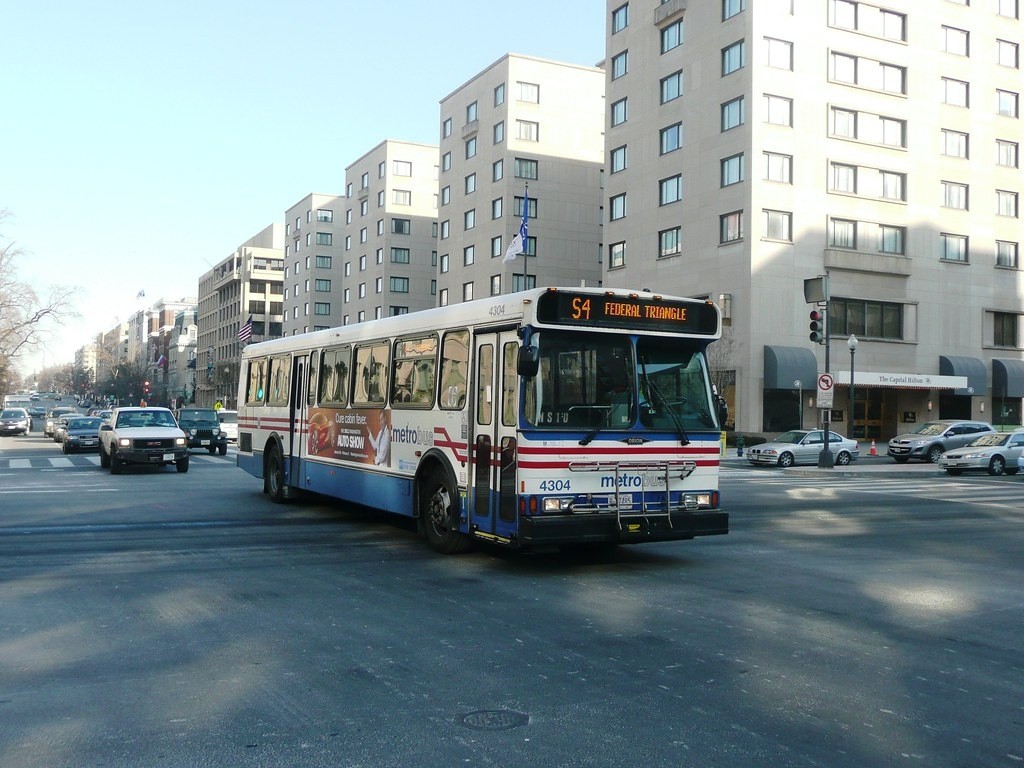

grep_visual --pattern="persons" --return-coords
[365,410,391,467]
[624,366,660,407]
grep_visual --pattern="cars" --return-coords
[64,418,106,452]
[746,429,859,468]
[888,419,998,462]
[0,409,31,436]
[216,410,238,443]
[3,388,109,443]
[939,426,1024,476]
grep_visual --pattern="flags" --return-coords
[238,313,252,344]
[502,195,527,264]
[157,355,166,367]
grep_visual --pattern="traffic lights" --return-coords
[809,310,824,343]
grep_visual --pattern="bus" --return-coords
[225,284,730,554]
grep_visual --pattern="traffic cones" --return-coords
[868,437,879,455]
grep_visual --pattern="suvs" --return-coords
[173,407,226,457]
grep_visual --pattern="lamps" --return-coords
[980,400,985,413]
[927,400,933,411]
[808,395,814,409]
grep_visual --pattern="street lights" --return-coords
[847,335,858,451]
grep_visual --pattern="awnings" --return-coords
[765,346,818,390]
[939,355,988,395]
[992,359,1024,396]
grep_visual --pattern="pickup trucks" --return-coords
[96,407,192,474]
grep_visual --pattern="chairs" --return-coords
[121,417,131,425]
[72,422,82,427]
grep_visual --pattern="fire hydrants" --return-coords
[736,431,745,457]
